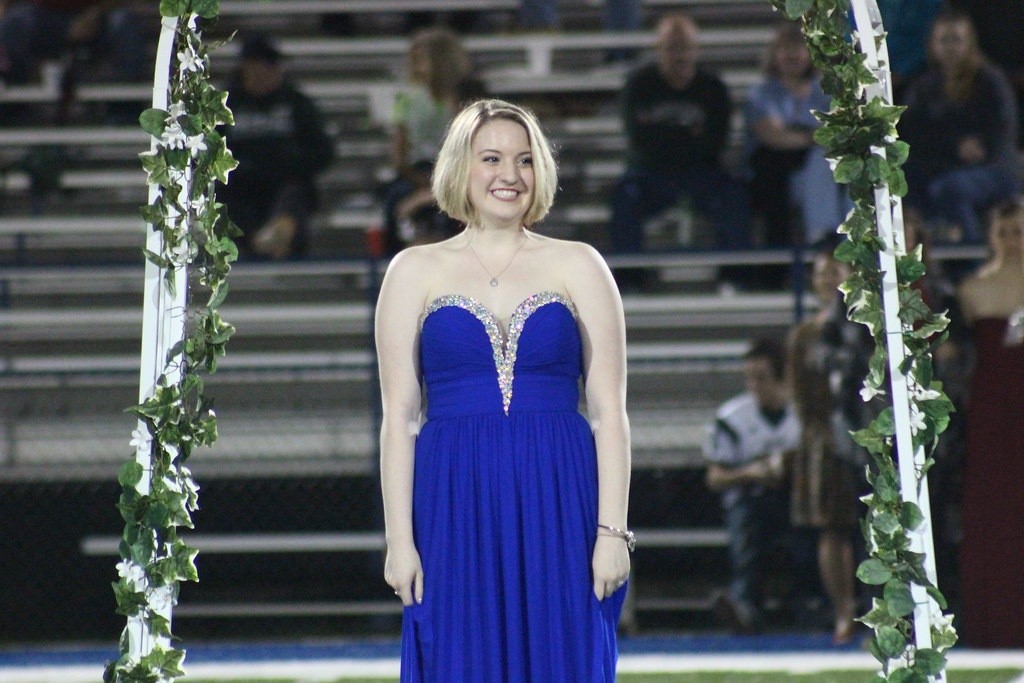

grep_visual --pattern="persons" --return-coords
[371,27,487,251]
[744,20,864,269]
[194,32,339,257]
[601,10,760,299]
[2,0,180,141]
[880,8,1024,250]
[700,200,1023,648]
[392,66,518,247]
[373,98,637,683]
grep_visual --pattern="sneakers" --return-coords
[718,281,742,298]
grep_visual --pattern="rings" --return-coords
[394,590,402,597]
[614,579,624,588]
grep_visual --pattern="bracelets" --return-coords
[589,514,635,557]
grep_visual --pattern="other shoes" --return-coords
[832,618,856,646]
[710,590,786,636]
[250,212,298,258]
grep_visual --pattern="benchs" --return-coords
[2,2,1021,634]
